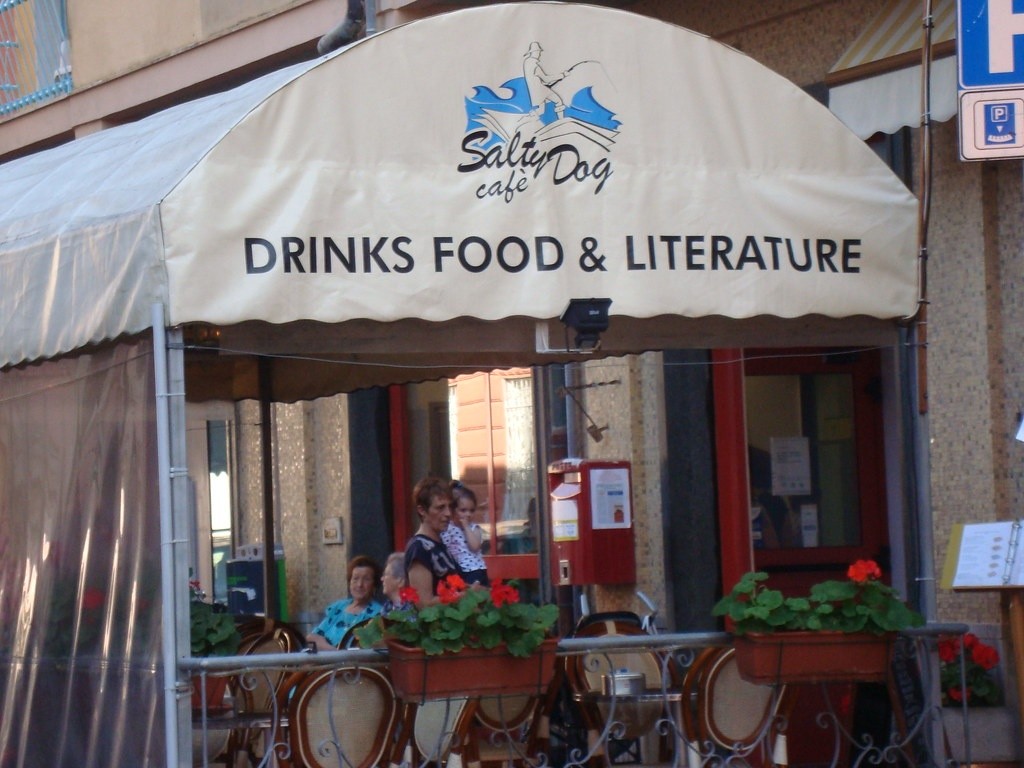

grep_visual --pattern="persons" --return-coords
[748,446,798,555]
[306,554,382,651]
[369,551,420,622]
[440,479,491,590]
[405,475,490,614]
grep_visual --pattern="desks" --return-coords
[188,707,287,768]
[572,683,700,768]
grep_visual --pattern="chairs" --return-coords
[462,694,550,768]
[567,608,673,767]
[680,646,786,768]
[289,666,400,768]
[211,628,297,768]
[407,698,470,768]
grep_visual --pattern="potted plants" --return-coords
[186,572,237,711]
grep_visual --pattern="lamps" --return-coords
[556,295,613,352]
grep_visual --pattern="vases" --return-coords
[384,626,562,705]
[729,609,891,684]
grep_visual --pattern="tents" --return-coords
[0,2,940,767]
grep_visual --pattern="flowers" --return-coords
[47,575,157,658]
[712,556,925,630]
[354,582,559,659]
[939,629,1000,709]
